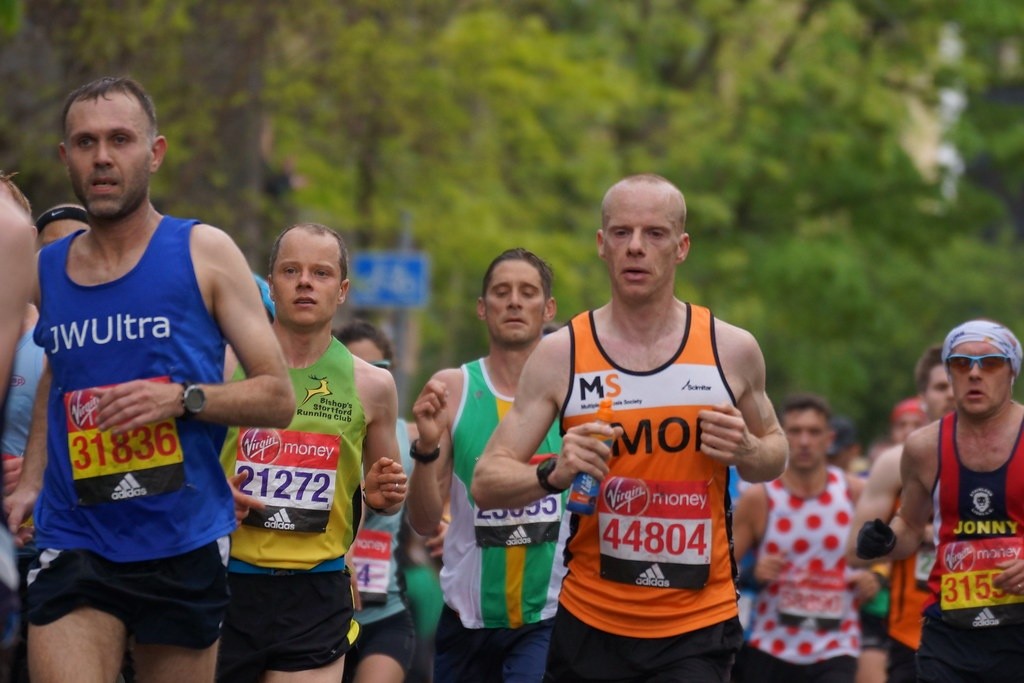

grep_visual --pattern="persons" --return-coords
[220,222,407,683]
[728,396,893,683]
[471,174,790,683]
[856,321,1024,683]
[398,246,573,683]
[335,321,450,683]
[828,346,958,683]
[0,77,296,683]
[1,175,89,683]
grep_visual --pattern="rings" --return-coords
[1018,584,1022,590]
[393,483,398,491]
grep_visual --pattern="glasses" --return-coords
[946,353,1011,373]
[370,360,391,370]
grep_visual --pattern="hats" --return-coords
[941,318,1022,386]
[888,397,929,424]
[828,417,856,456]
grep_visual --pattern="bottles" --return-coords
[565,399,615,515]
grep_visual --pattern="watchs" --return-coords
[410,438,440,463]
[537,456,565,494]
[177,382,206,421]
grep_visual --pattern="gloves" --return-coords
[856,518,897,561]
